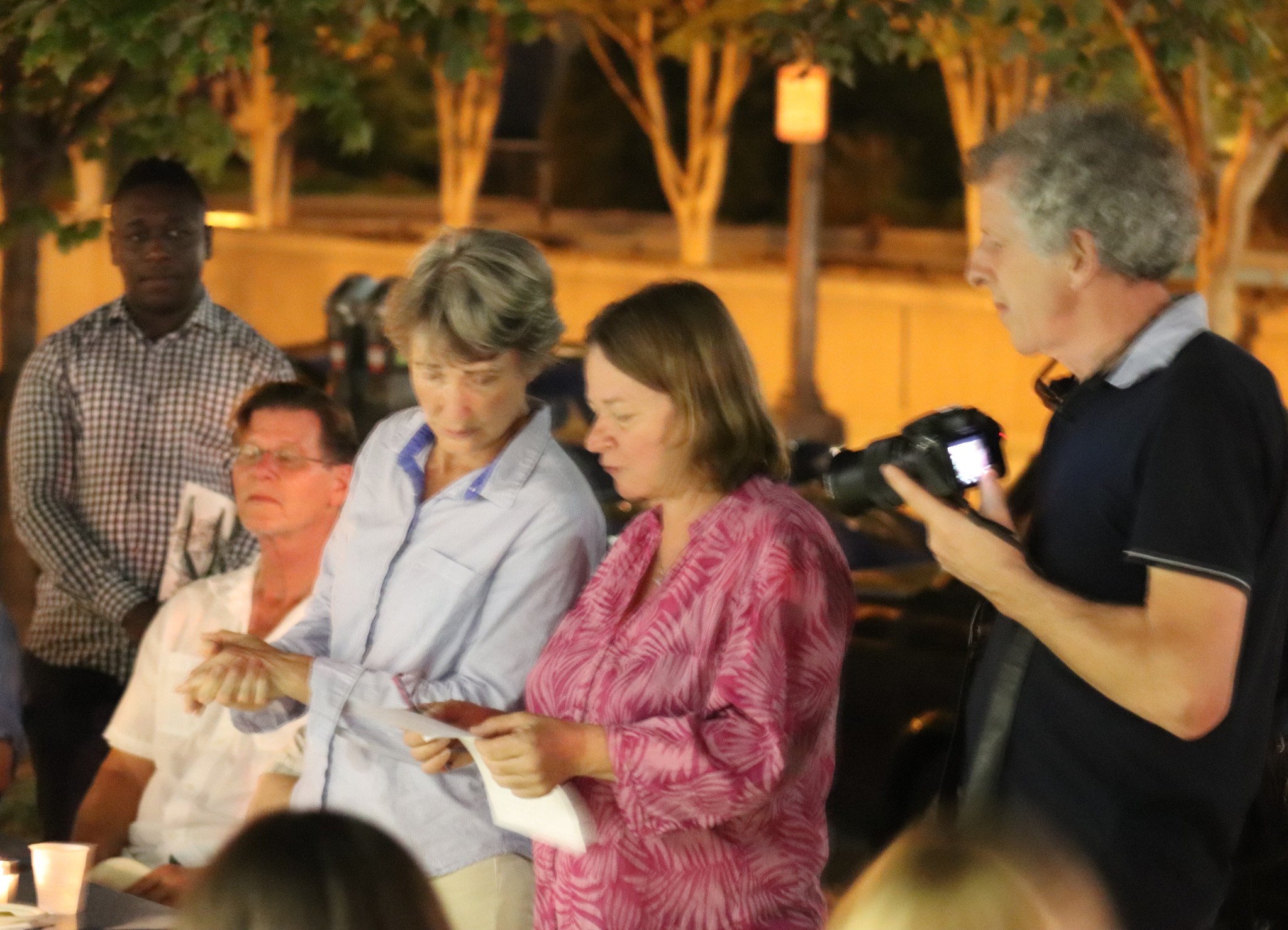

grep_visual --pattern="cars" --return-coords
[271,342,615,498]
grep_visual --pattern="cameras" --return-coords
[826,409,1008,517]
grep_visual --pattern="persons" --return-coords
[880,90,1288,930]
[4,154,289,848]
[0,225,851,930]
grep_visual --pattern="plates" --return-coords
[0,902,55,930]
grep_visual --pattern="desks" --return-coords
[0,869,176,930]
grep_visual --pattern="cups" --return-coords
[28,841,90,916]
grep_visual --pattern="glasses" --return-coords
[235,444,327,473]
